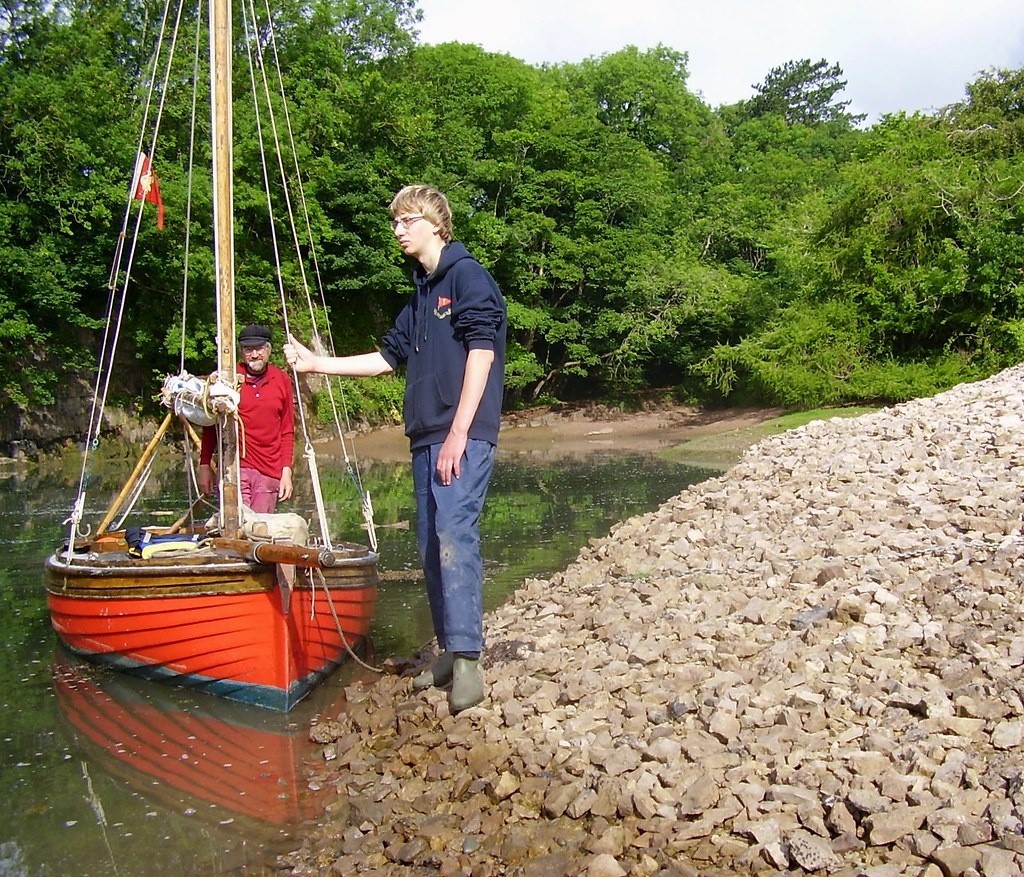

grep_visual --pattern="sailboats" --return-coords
[42,1,382,712]
[42,635,378,841]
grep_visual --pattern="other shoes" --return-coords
[451,653,484,710]
[412,649,453,689]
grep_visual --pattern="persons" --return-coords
[283,186,507,712]
[200,325,296,518]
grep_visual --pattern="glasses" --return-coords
[392,216,424,229]
[242,343,267,355]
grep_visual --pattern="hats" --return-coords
[238,325,270,347]
